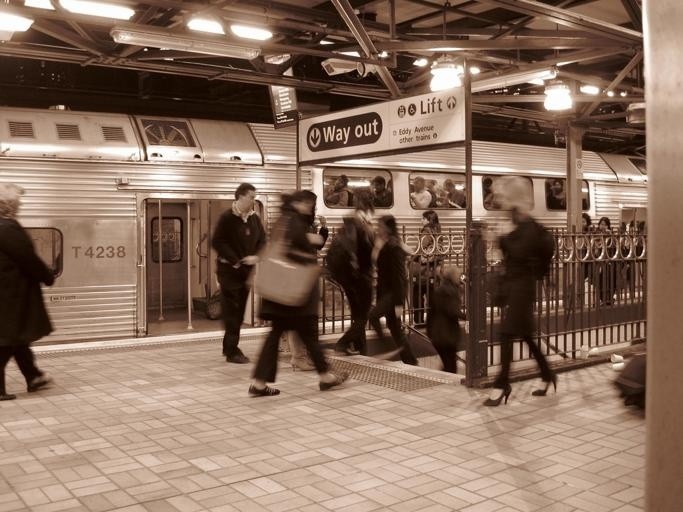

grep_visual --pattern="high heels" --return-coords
[531,374,557,397]
[483,383,513,407]
[290,357,317,372]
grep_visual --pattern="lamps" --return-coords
[0,0,274,61]
[544,81,573,111]
[471,67,557,94]
[429,60,462,93]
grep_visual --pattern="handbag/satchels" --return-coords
[246,213,322,307]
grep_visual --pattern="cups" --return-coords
[579,346,590,361]
[610,355,624,363]
[589,348,599,358]
[612,363,625,371]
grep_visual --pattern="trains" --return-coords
[0,106,649,346]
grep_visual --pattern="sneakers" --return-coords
[248,383,281,397]
[27,376,54,392]
[334,343,360,356]
[0,393,17,401]
[320,372,350,391]
[226,352,250,364]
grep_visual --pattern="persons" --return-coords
[582,213,647,305]
[211,183,266,364]
[0,182,57,401]
[247,174,566,409]
